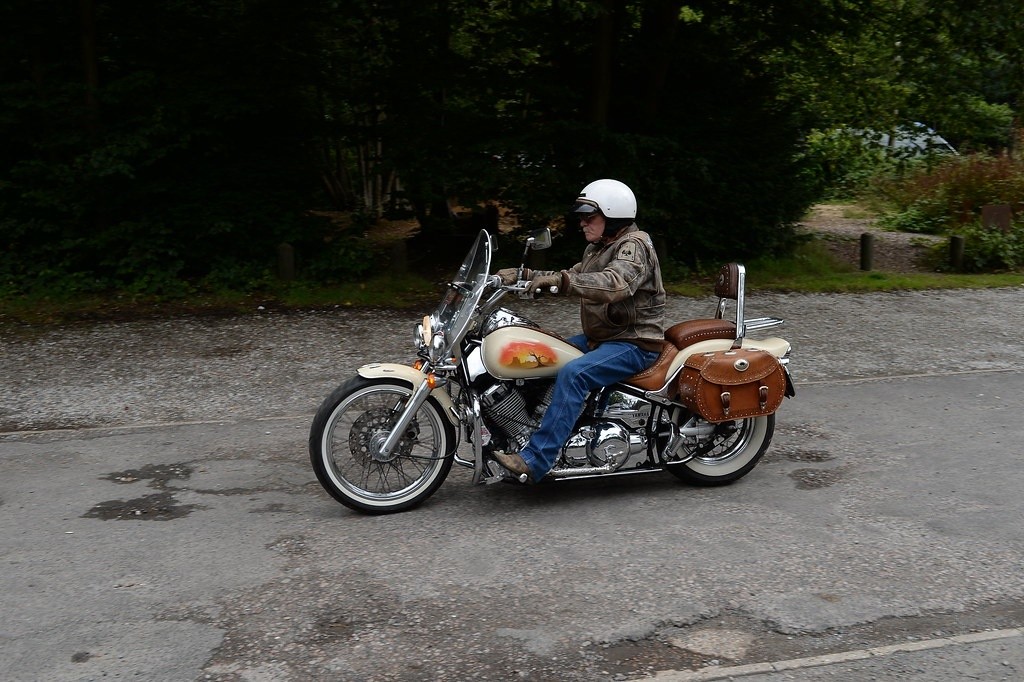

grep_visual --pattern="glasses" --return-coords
[580,213,599,222]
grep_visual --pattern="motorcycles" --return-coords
[308,225,793,516]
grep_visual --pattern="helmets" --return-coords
[573,178,638,221]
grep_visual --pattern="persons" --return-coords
[495,179,666,483]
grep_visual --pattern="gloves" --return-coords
[494,267,521,285]
[522,275,562,298]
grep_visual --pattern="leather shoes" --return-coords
[490,449,531,478]
